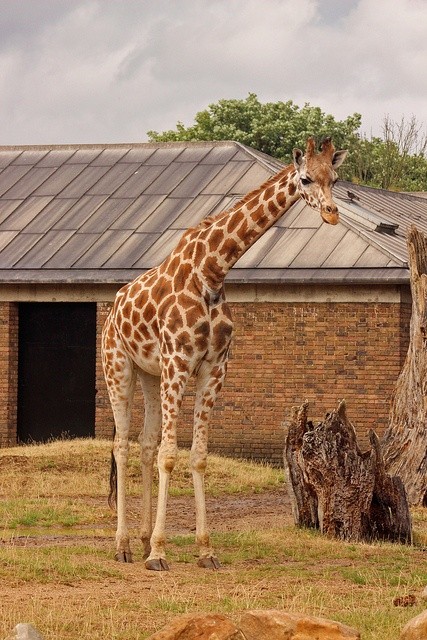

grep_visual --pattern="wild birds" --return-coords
[346,190,361,202]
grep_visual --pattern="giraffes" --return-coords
[100,134,349,574]
